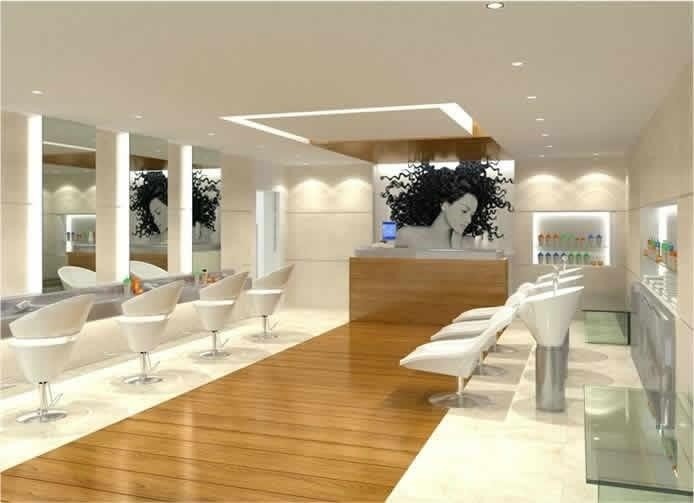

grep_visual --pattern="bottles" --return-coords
[641,229,678,274]
[536,230,605,266]
[124,278,132,297]
[201,269,208,286]
[63,230,95,242]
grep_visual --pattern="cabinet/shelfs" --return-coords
[630,281,677,429]
[348,256,510,325]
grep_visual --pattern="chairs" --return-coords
[193,272,249,360]
[57,265,96,289]
[431,292,525,376]
[399,308,513,409]
[453,283,534,353]
[8,293,97,424]
[246,264,295,344]
[114,280,184,385]
[129,261,168,280]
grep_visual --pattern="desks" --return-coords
[584,383,694,503]
[580,288,631,345]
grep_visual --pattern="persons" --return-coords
[129,169,222,245]
[381,159,515,250]
